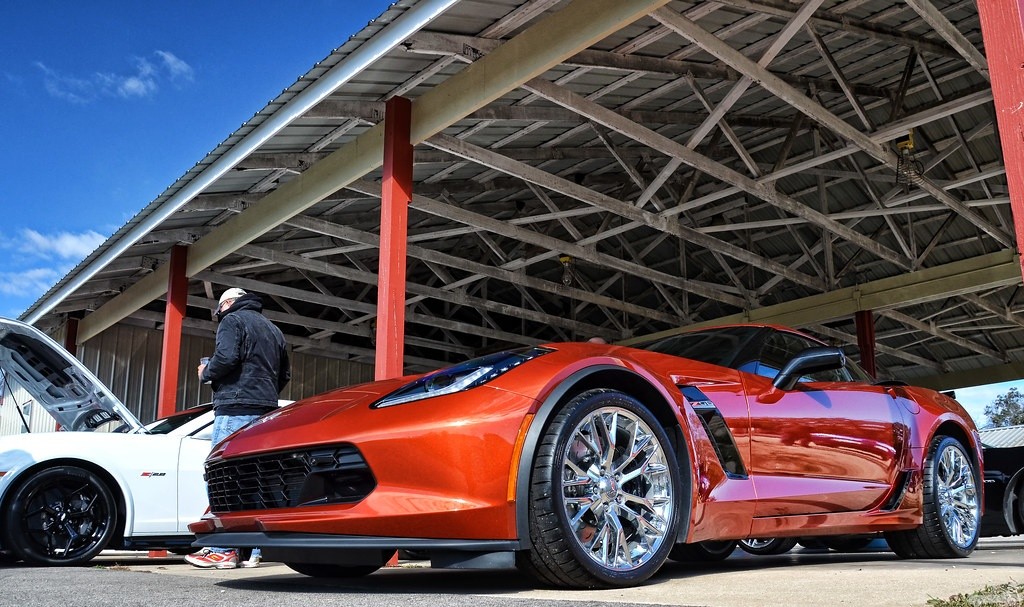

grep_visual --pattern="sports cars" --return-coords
[187,323,985,588]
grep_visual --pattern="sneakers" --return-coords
[236,554,260,568]
[185,546,239,569]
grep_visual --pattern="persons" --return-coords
[184,289,292,569]
[589,338,606,344]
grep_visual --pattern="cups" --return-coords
[200,357,212,384]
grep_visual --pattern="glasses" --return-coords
[218,301,226,314]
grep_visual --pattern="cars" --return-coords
[0,316,296,567]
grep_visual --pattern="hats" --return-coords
[212,288,247,315]
